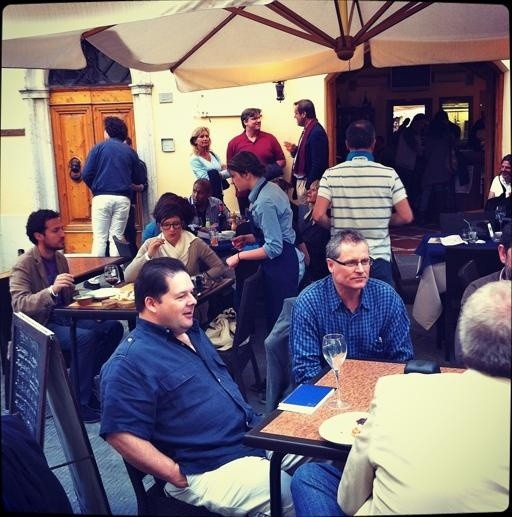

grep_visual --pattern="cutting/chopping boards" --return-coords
[102,282,136,309]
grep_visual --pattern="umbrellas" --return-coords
[2,0,511,93]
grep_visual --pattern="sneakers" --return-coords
[248,378,267,404]
[87,393,104,412]
[80,405,102,423]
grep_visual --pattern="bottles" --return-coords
[206,217,211,230]
[230,210,237,230]
[217,203,226,232]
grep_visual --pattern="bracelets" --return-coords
[237,252,241,260]
[50,285,59,296]
[202,272,208,279]
[205,271,212,278]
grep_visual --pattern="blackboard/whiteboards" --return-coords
[8,314,51,452]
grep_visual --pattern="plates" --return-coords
[88,287,119,299]
[318,411,370,445]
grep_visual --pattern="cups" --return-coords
[208,230,219,246]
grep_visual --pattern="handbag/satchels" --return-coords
[205,308,250,351]
[446,143,461,175]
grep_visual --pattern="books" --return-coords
[277,382,337,415]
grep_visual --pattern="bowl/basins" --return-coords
[221,230,233,238]
[73,294,93,306]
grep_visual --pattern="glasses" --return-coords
[251,114,262,121]
[325,256,375,268]
[160,221,182,230]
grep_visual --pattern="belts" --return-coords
[295,176,305,180]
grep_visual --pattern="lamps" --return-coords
[273,79,288,101]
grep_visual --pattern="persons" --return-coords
[185,177,234,233]
[269,176,297,231]
[99,257,295,516]
[226,108,287,218]
[9,209,123,424]
[414,110,460,225]
[81,116,138,290]
[483,153,511,219]
[393,114,422,209]
[289,277,511,517]
[142,190,200,248]
[452,226,511,371]
[397,118,410,134]
[310,117,415,292]
[282,226,414,401]
[292,179,329,294]
[283,100,330,204]
[124,199,229,284]
[222,150,300,404]
[188,124,231,200]
[120,137,148,262]
[392,117,399,128]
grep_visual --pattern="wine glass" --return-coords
[104,264,120,287]
[322,333,351,409]
